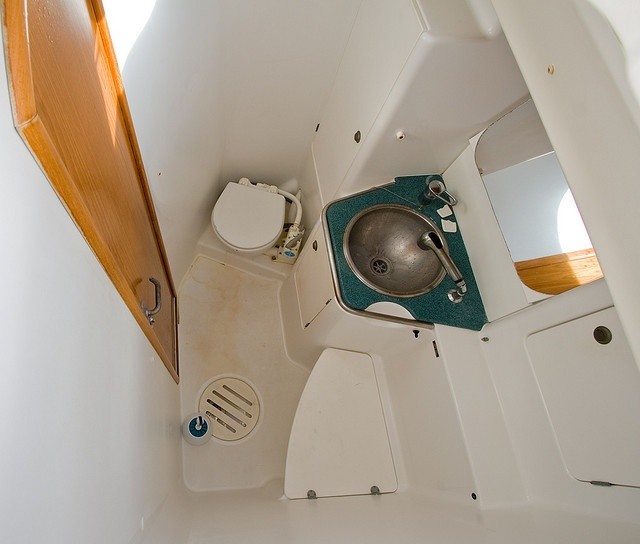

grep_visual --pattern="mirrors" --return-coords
[474,98,603,295]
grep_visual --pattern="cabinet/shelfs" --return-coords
[310,1,532,210]
[292,217,335,329]
[525,306,640,488]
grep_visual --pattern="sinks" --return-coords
[341,203,450,299]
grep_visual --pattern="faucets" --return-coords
[416,230,468,304]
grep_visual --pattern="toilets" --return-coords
[210,177,287,254]
